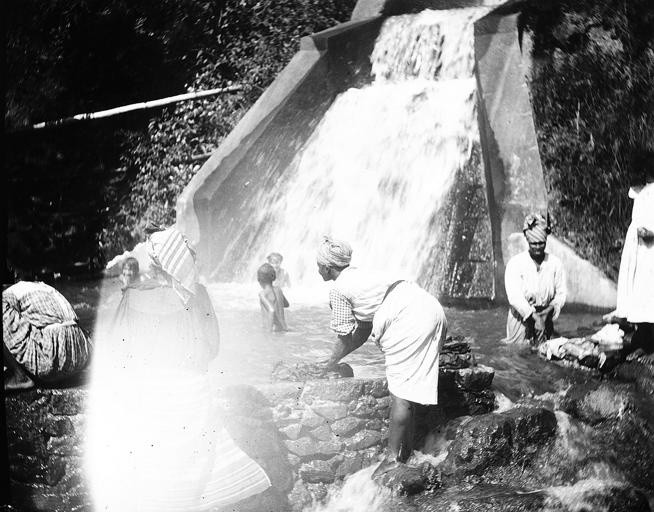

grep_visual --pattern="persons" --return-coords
[267,252,291,285]
[116,257,154,290]
[258,262,292,333]
[503,210,568,346]
[0,267,90,392]
[307,234,452,465]
[111,220,222,378]
